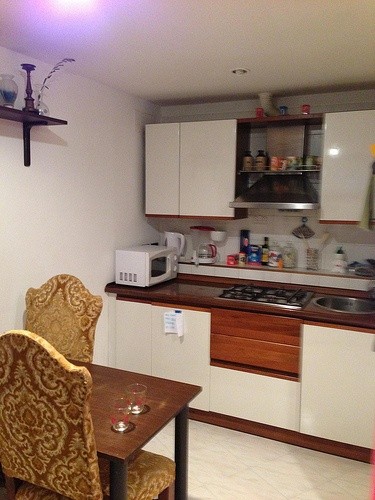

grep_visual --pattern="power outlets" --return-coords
[251,216,268,225]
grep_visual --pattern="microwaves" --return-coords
[114,246,178,287]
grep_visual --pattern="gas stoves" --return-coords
[215,284,314,310]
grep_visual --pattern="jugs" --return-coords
[196,243,217,259]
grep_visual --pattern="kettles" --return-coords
[164,231,186,257]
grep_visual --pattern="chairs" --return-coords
[0,329,176,500]
[24,273,103,362]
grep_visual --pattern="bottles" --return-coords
[267,241,281,268]
[239,252,246,264]
[243,150,252,171]
[331,246,347,274]
[255,150,266,171]
[261,236,270,265]
[282,241,298,268]
[270,153,278,171]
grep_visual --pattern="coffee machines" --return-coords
[189,225,226,264]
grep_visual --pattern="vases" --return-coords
[0,74,19,107]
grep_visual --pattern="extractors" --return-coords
[229,125,319,210]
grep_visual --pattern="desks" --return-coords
[60,354,201,500]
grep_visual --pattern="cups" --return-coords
[256,107,264,117]
[305,155,318,169]
[287,156,296,167]
[306,247,318,271]
[300,104,310,114]
[108,395,135,432]
[279,106,288,115]
[123,383,147,415]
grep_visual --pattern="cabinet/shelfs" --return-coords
[149,304,212,423]
[298,321,375,461]
[318,110,375,225]
[108,295,151,377]
[240,112,323,175]
[143,117,248,220]
